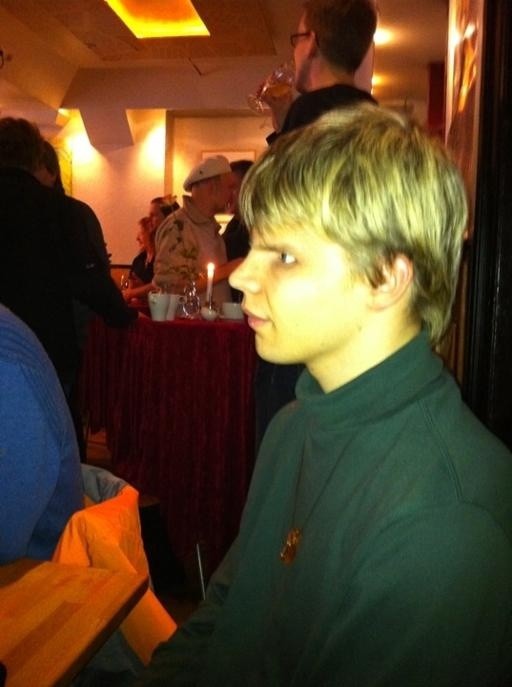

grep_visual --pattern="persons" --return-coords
[241,1,379,146]
[153,151,247,308]
[127,214,150,284]
[1,115,132,462]
[122,195,179,306]
[113,98,512,686]
[32,133,66,198]
[223,157,256,305]
[0,306,93,686]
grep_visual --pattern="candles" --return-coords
[205,260,217,299]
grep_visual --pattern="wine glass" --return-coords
[244,55,294,113]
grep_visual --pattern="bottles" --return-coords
[177,271,201,318]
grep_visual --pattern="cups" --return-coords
[150,293,186,321]
[119,273,132,289]
[217,302,243,319]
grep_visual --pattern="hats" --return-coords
[182,154,233,192]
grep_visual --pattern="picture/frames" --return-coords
[160,106,279,224]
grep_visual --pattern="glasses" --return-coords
[289,31,310,47]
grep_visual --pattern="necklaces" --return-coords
[276,412,356,570]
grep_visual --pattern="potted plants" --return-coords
[159,207,201,319]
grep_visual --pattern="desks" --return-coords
[86,310,260,565]
